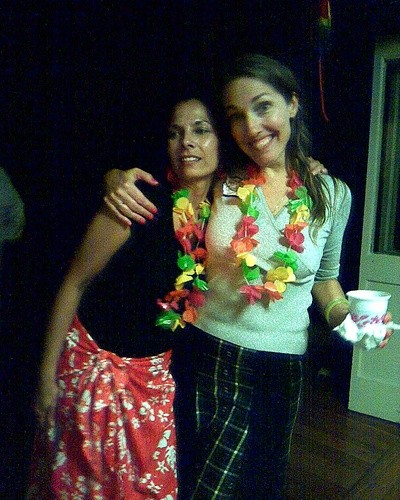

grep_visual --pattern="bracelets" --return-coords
[324,298,350,324]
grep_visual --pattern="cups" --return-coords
[346,290,392,331]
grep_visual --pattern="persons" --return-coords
[27,89,327,500]
[104,53,392,500]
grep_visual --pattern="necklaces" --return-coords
[237,161,313,308]
[155,170,213,333]
